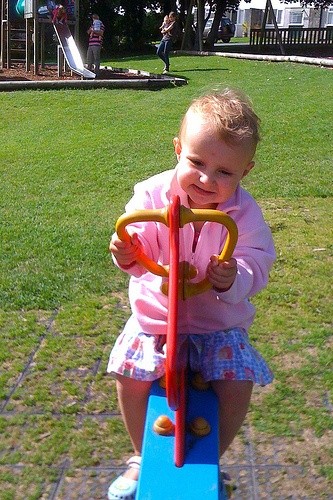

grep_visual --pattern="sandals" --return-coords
[107,454,142,500]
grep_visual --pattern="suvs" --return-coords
[197,18,237,43]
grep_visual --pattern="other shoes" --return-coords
[162,70,168,74]
[163,68,165,70]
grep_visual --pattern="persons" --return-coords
[86,22,104,75]
[90,15,102,40]
[52,7,67,25]
[156,11,177,74]
[107,87,277,500]
[159,15,172,39]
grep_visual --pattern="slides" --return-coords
[51,17,97,79]
